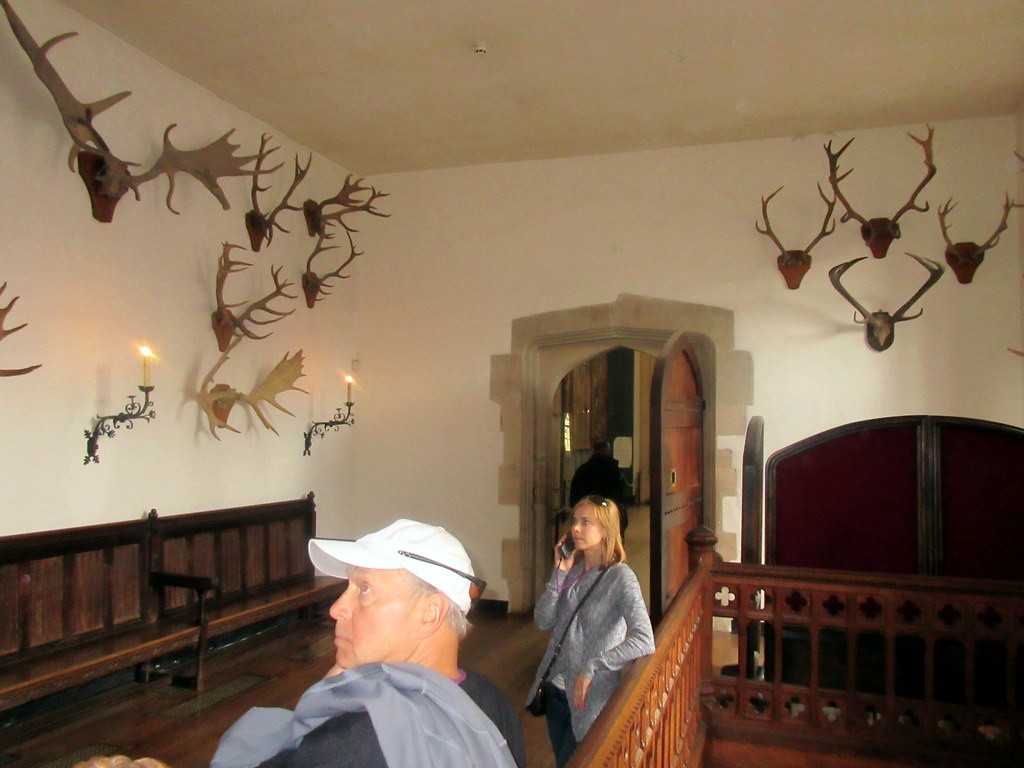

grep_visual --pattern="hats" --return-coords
[308,518,476,615]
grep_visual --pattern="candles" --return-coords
[142,347,152,386]
[345,376,353,403]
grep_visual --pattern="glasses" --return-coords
[399,550,487,602]
[581,495,608,507]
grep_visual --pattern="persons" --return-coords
[569,439,633,553]
[522,493,655,768]
[67,518,527,767]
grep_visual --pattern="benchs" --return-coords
[0,489,351,713]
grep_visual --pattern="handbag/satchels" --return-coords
[526,677,546,717]
